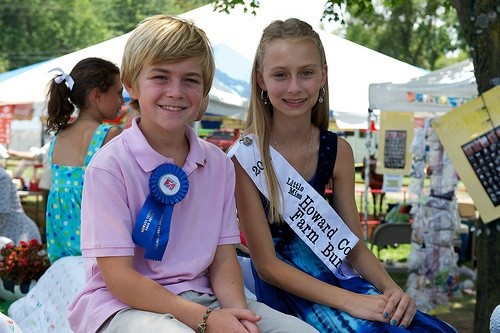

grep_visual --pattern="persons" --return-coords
[227,18,461,333]
[66,13,321,332]
[46,57,124,264]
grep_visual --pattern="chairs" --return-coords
[371,223,413,274]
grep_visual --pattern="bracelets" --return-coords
[196,306,214,333]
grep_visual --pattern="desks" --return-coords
[355,189,385,219]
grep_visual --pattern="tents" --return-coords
[365,55,480,241]
[0,0,429,131]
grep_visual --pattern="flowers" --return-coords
[0,237,50,284]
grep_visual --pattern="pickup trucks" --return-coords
[206,128,240,152]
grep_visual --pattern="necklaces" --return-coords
[269,125,313,178]
